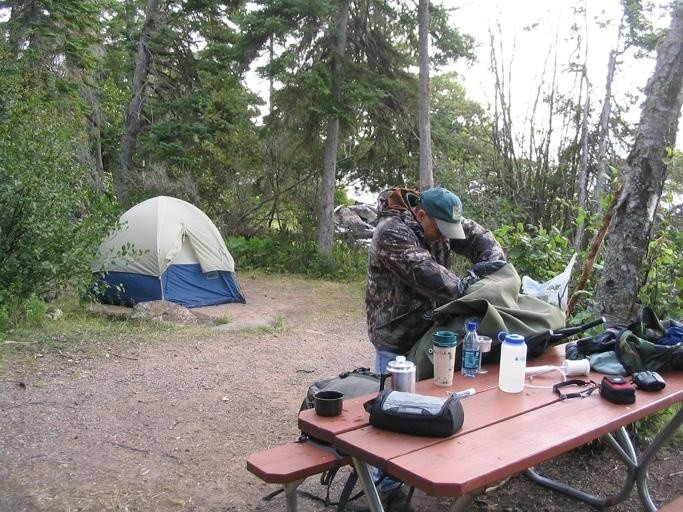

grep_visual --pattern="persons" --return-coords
[365,183,507,512]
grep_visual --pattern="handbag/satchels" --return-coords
[408,263,567,384]
[599,372,638,406]
[361,388,464,439]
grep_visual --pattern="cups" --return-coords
[478,335,493,374]
[314,391,343,415]
[432,330,459,387]
[380,355,417,394]
[497,331,528,394]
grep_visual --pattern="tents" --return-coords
[79,194,247,309]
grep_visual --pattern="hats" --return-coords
[420,187,467,240]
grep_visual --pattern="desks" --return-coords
[299,336,683,512]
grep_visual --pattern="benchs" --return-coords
[246,436,360,511]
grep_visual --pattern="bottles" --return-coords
[461,322,481,378]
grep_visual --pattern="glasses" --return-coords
[428,216,443,236]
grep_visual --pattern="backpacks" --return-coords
[294,367,388,446]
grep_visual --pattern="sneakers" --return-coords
[376,487,415,511]
